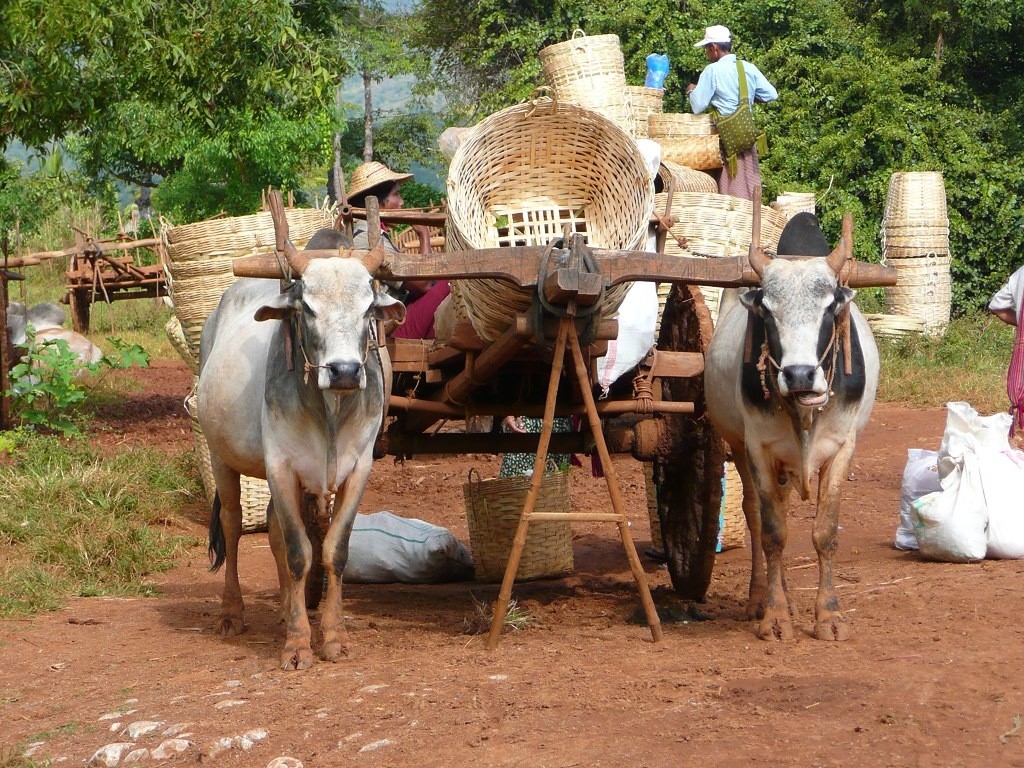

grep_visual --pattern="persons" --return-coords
[684,25,777,201]
[987,265,1024,449]
[346,160,452,340]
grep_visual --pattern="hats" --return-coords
[337,161,414,206]
[693,25,732,47]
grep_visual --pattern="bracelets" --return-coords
[686,90,693,95]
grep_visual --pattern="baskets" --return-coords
[445,28,789,346]
[160,208,333,375]
[865,312,924,343]
[881,171,953,339]
[643,457,747,555]
[463,459,575,581]
[188,394,271,535]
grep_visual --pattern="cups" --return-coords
[646,48,667,88]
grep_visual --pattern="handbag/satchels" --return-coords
[893,449,943,549]
[714,104,767,161]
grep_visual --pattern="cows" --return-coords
[193,255,408,674]
[703,236,882,643]
